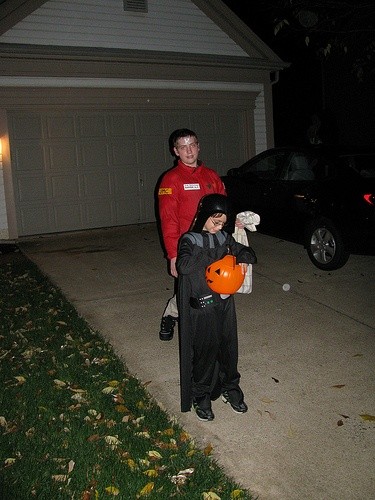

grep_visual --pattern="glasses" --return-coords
[177,141,198,150]
[210,217,225,226]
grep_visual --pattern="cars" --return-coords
[219,139,375,271]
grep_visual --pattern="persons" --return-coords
[176,193,258,421]
[158,129,244,341]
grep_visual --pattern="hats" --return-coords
[194,194,231,233]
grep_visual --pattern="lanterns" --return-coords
[205,256,245,295]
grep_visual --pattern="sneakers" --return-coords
[159,317,176,341]
[222,388,248,414]
[193,398,214,421]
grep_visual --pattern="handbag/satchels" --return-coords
[232,211,261,294]
